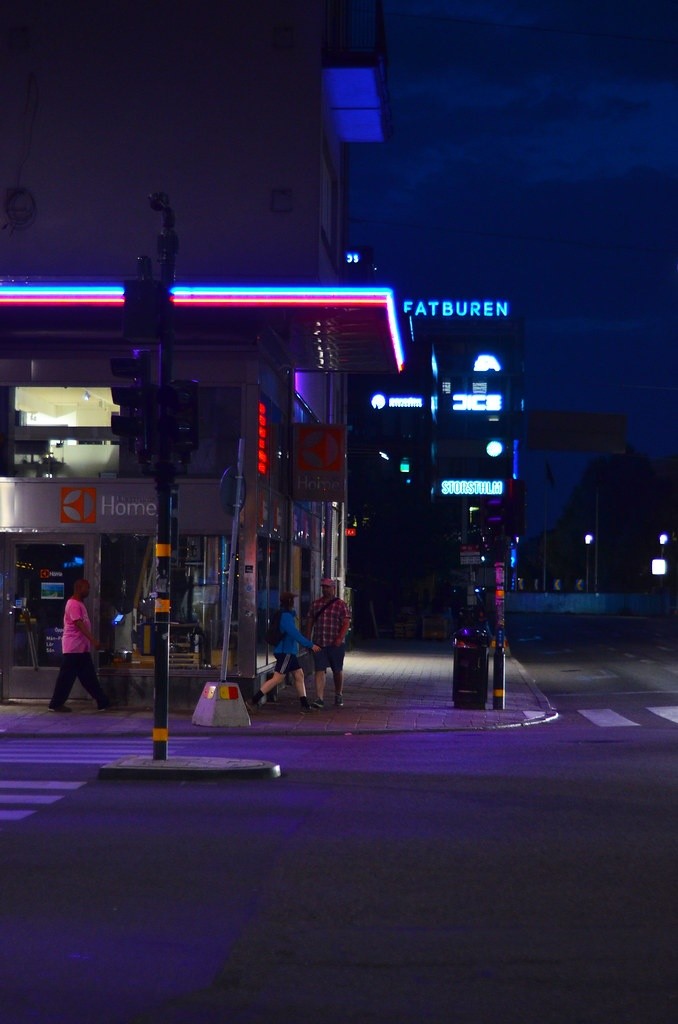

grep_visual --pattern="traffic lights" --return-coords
[110,347,155,454]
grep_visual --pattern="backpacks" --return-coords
[264,610,290,646]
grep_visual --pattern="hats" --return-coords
[280,592,299,601]
[320,579,335,586]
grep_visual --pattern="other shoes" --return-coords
[312,697,324,708]
[247,699,260,716]
[334,694,344,705]
[300,706,320,714]
[48,704,72,712]
[99,697,121,711]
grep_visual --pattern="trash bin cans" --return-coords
[451,626,493,711]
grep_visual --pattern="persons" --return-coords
[48,579,119,712]
[244,592,323,714]
[305,578,350,710]
